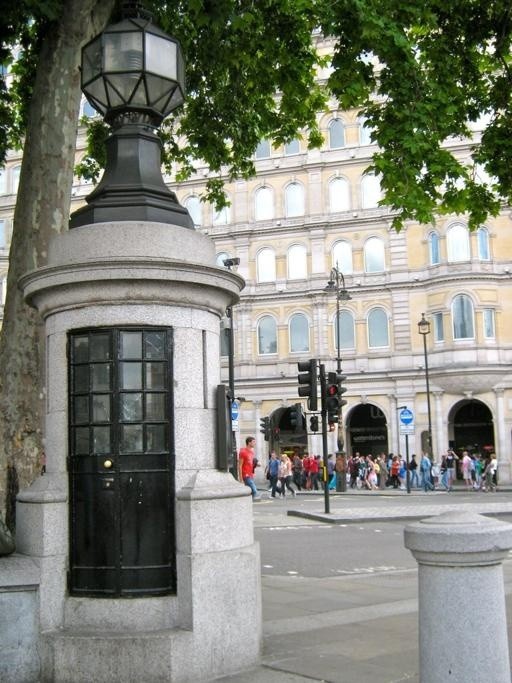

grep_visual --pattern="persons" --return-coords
[263,448,499,500]
[236,436,262,501]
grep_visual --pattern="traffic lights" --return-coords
[274,415,280,442]
[260,415,268,441]
[290,355,351,436]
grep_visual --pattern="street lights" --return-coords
[325,257,352,494]
[418,309,435,469]
